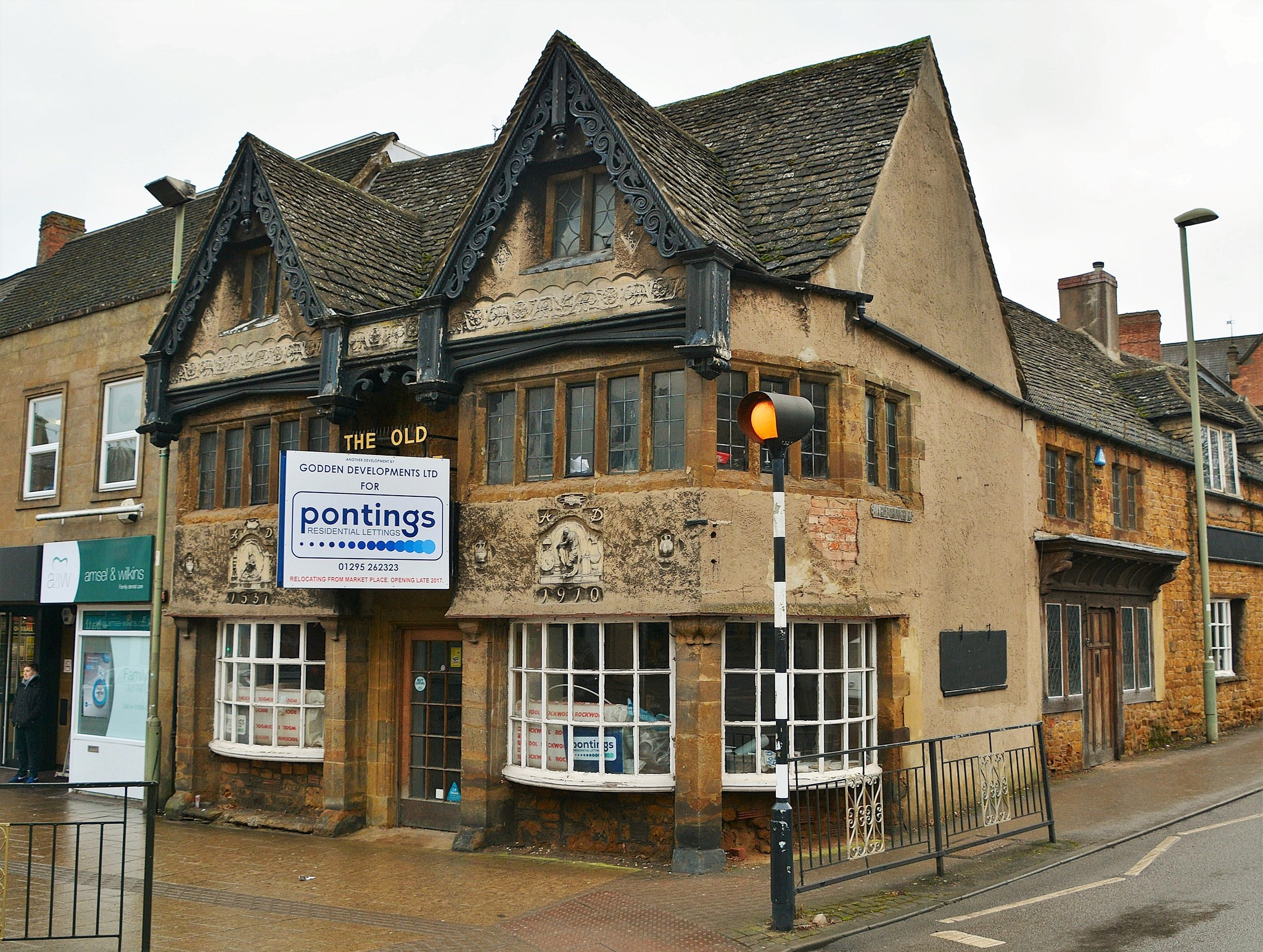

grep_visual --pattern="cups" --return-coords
[795,752,801,762]
[734,734,769,755]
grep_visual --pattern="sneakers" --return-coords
[8,775,28,783]
[25,776,39,783]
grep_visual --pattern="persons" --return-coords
[8,664,45,784]
[244,553,255,573]
[558,527,579,563]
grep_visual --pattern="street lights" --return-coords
[1175,207,1220,744]
[739,390,814,933]
